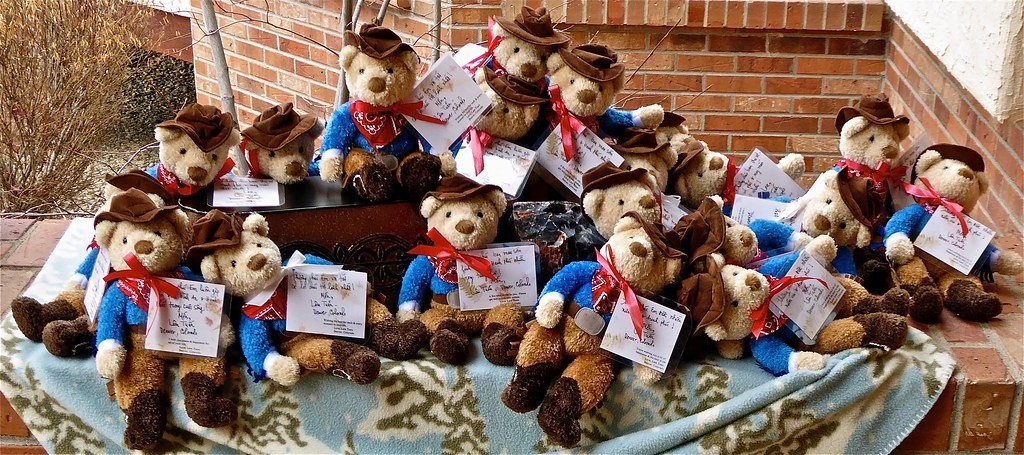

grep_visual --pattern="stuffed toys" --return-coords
[397,176,529,367]
[11,4,1023,453]
